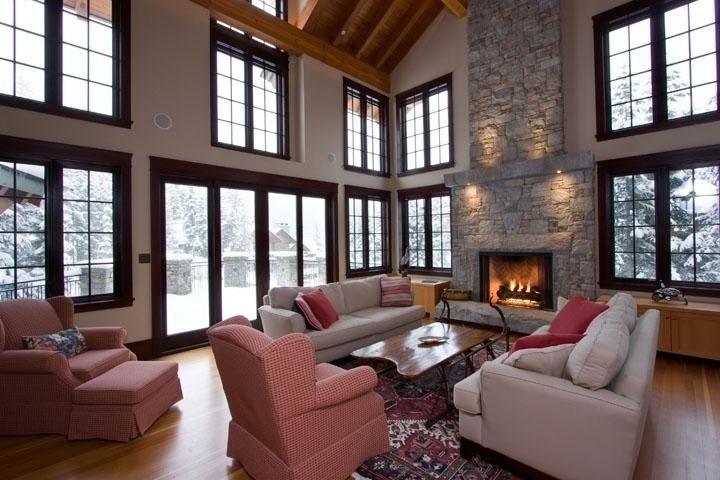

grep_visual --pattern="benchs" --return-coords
[68,359,185,443]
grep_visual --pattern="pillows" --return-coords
[379,276,413,308]
[21,326,86,362]
[293,287,340,332]
[501,293,609,377]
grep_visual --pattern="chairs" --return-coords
[205,314,391,478]
[1,294,138,436]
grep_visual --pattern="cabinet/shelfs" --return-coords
[595,294,720,363]
[409,279,450,320]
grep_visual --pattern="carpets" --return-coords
[327,339,520,479]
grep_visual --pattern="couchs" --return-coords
[256,272,426,366]
[452,293,662,480]
